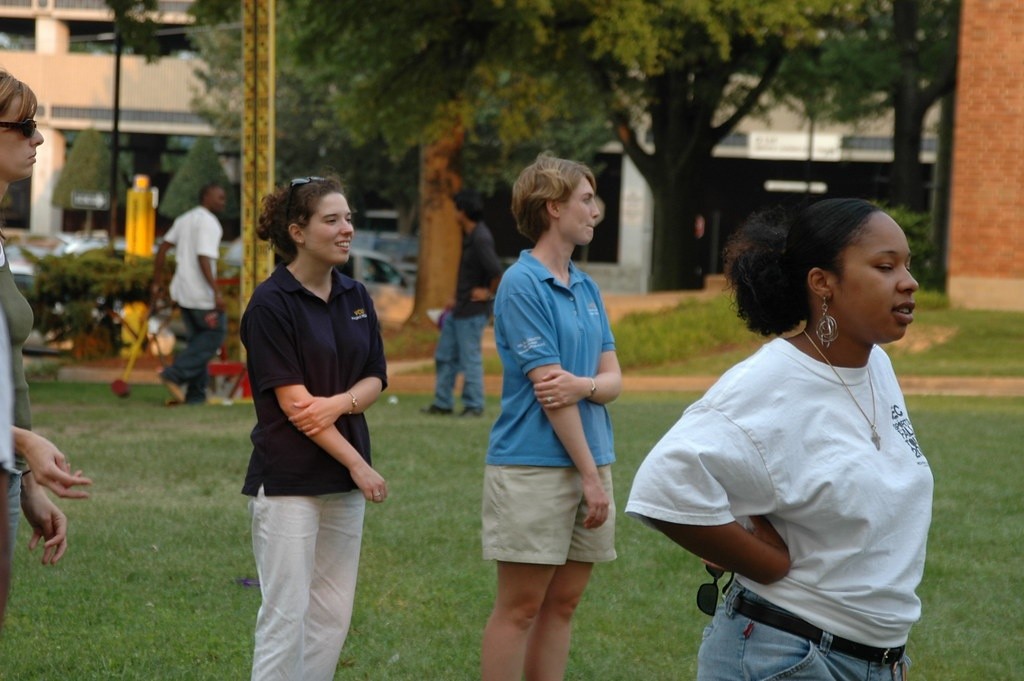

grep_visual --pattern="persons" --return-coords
[148,183,233,404]
[482,155,624,681]
[239,175,388,681]
[1,70,92,621]
[420,188,504,417]
[624,199,936,681]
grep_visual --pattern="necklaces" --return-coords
[801,328,882,453]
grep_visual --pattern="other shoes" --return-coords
[186,392,224,404]
[159,370,186,402]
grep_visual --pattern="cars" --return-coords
[340,249,416,328]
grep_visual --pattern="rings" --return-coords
[548,395,553,403]
[373,493,381,498]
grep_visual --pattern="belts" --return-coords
[736,593,905,666]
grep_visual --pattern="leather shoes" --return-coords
[421,404,482,417]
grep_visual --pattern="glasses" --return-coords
[697,563,734,616]
[287,177,326,221]
[0,119,36,138]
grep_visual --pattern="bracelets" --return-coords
[587,376,597,401]
[21,469,32,476]
[346,391,358,415]
